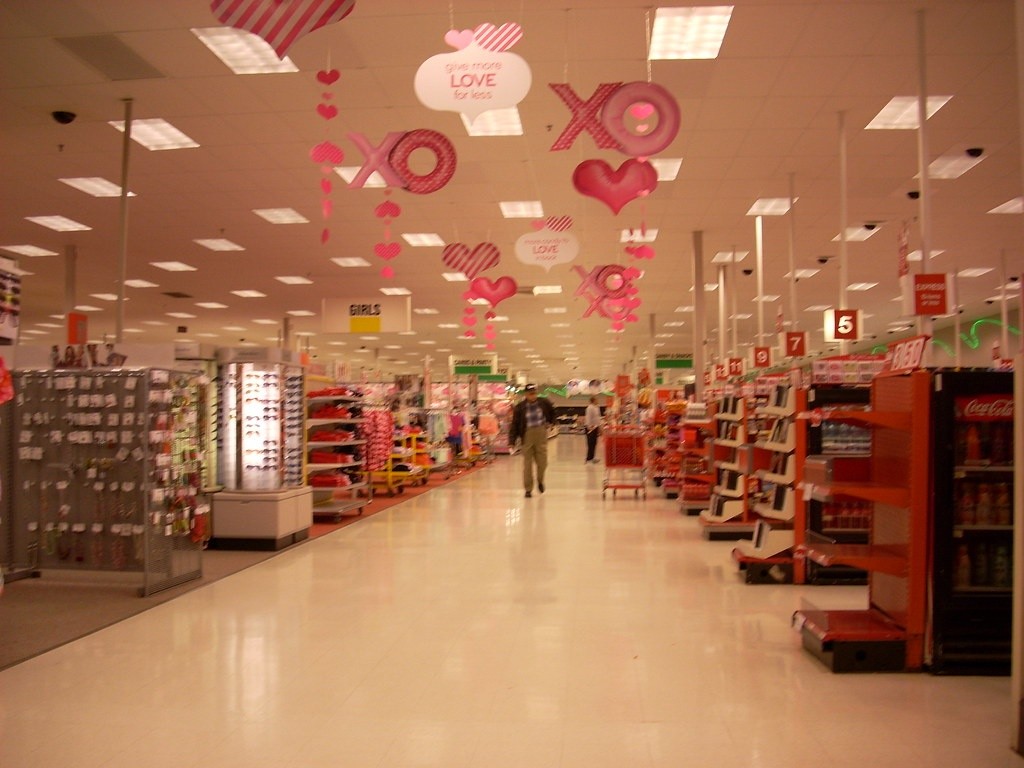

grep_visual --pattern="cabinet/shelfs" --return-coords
[635,355,1024,755]
[0,346,494,597]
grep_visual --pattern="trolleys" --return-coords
[600,424,650,501]
[343,433,431,497]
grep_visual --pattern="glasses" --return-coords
[210,362,305,489]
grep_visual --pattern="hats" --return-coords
[525,384,538,392]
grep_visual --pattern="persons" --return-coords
[507,382,556,498]
[584,397,605,463]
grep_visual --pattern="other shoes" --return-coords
[539,483,544,493]
[525,492,531,497]
